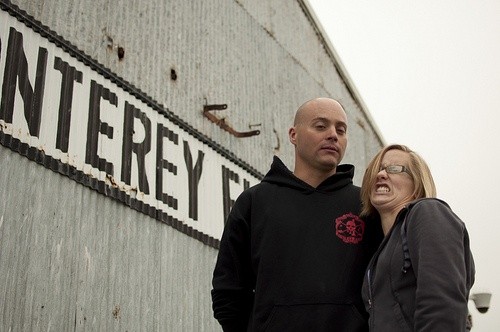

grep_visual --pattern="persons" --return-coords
[212,98,383,332]
[360,145,475,332]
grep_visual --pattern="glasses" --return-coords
[378,165,413,176]
